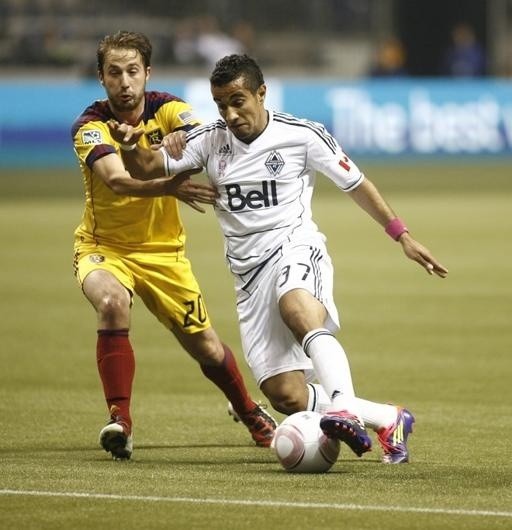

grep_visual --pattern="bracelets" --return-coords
[385,217,409,242]
[119,143,136,151]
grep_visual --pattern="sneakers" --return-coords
[226,402,277,448]
[378,403,414,465]
[320,410,372,458]
[98,415,134,458]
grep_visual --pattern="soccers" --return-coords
[275,411,341,473]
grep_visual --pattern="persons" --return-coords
[1,1,498,78]
[106,52,448,464]
[71,31,279,461]
